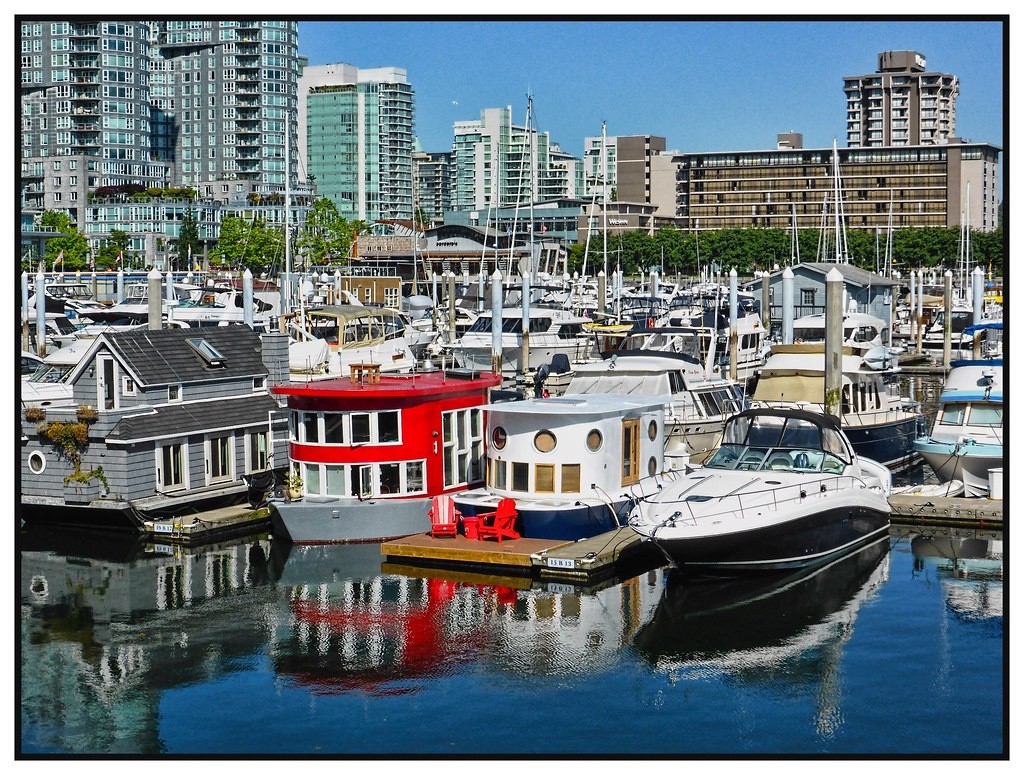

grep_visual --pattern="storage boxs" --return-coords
[461,516,484,540]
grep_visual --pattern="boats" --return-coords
[16,87,1002,588]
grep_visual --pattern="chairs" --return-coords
[426,495,463,538]
[476,498,522,544]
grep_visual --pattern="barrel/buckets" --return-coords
[462,515,485,539]
